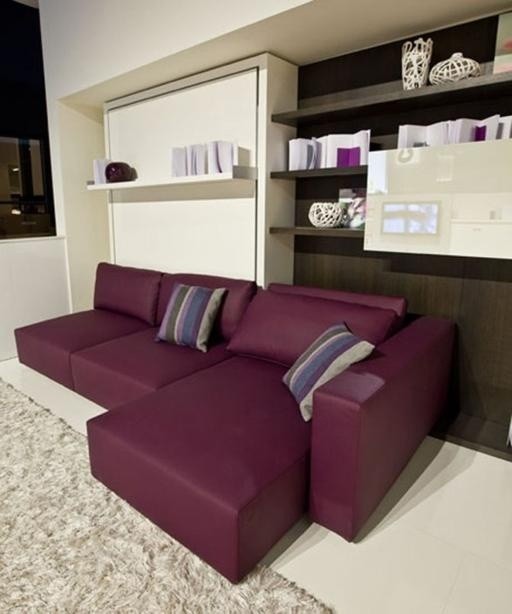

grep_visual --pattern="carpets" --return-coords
[0,378,333,614]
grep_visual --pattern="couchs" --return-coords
[12,262,454,582]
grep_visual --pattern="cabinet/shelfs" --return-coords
[269,71,512,236]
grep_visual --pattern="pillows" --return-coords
[226,288,396,367]
[282,320,375,421]
[155,283,226,353]
